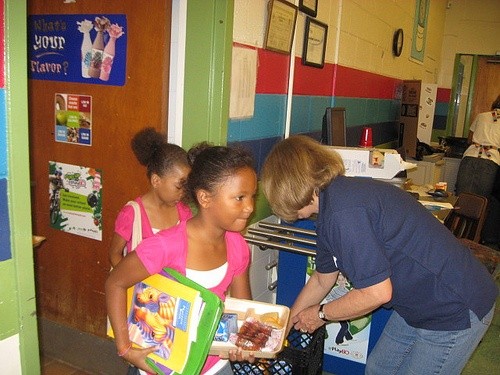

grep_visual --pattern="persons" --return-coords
[105,143,257,375]
[455,95,500,243]
[110,127,192,375]
[260,136,496,375]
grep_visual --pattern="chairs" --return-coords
[450,192,487,244]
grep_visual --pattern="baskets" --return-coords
[226,314,326,375]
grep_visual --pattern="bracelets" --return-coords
[117,343,131,357]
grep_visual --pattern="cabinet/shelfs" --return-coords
[405,157,462,193]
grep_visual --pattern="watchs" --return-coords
[319,304,331,322]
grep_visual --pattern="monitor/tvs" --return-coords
[321,107,346,147]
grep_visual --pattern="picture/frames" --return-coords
[395,29,403,56]
[298,0,319,17]
[302,16,328,67]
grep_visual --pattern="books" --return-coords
[106,267,225,375]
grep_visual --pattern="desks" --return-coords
[275,194,461,375]
